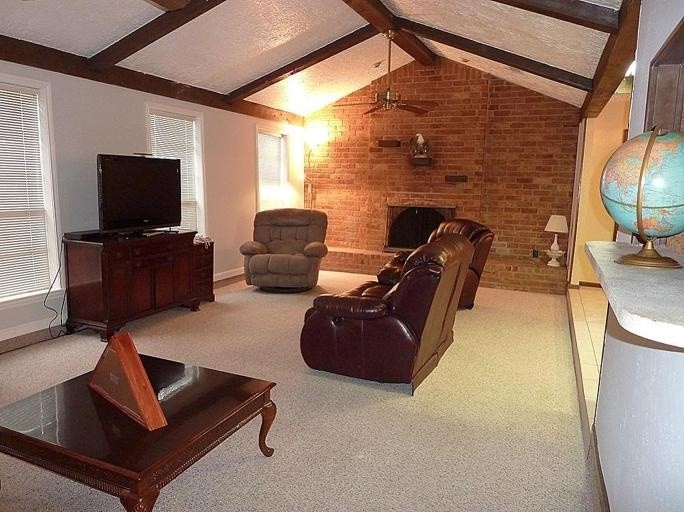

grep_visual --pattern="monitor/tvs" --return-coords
[98,153,182,231]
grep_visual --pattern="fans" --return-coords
[331,29,439,115]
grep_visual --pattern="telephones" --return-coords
[195,233,210,243]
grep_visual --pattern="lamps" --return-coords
[544,215,568,250]
[412,132,428,158]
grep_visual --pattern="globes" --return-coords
[599,123,684,268]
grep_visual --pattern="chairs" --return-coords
[378,219,494,310]
[239,208,328,293]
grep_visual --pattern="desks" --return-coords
[1,353,276,512]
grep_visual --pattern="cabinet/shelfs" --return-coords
[192,240,214,303]
[63,229,198,342]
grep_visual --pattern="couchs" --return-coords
[301,233,475,395]
